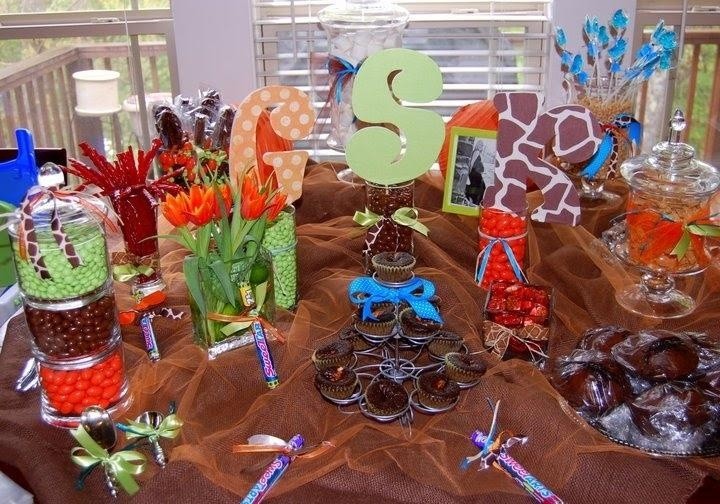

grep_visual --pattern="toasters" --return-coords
[316,0,410,184]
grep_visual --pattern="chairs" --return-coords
[0,148,68,193]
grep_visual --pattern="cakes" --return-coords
[312,250,482,418]
[554,325,720,453]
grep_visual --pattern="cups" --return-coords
[107,173,168,294]
[478,197,528,291]
[618,107,719,263]
[238,206,300,312]
[364,176,414,256]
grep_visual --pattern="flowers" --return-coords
[138,161,290,312]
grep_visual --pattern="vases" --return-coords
[185,243,280,362]
[565,73,641,180]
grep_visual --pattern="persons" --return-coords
[466,140,486,205]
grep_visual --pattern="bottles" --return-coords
[9,161,135,430]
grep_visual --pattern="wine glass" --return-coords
[563,71,637,209]
[601,223,707,318]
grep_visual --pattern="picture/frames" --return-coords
[441,125,500,218]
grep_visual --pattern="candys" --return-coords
[367,185,415,278]
[151,90,236,183]
[261,210,299,312]
[11,222,125,417]
[554,8,679,103]
[488,280,554,360]
[476,208,530,292]
[630,189,710,274]
